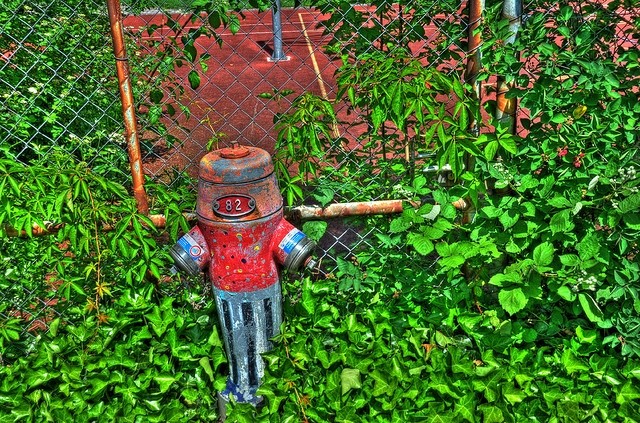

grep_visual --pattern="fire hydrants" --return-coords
[169,141,317,422]
